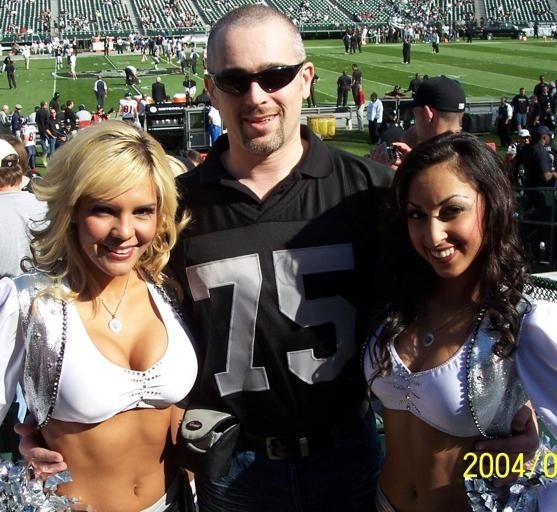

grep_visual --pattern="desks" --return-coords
[381,98,414,123]
[91,41,113,53]
[306,115,335,140]
[461,110,493,132]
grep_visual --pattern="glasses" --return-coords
[206,61,304,95]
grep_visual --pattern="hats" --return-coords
[15,104,23,109]
[517,125,554,138]
[397,78,465,112]
[0,138,19,167]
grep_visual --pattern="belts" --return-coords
[244,387,371,460]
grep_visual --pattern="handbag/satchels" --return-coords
[180,408,241,484]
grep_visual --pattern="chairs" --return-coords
[0,0,554,37]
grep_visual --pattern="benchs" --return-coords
[35,44,73,53]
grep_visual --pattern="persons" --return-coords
[0,0,557,512]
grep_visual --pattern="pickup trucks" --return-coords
[475,22,526,40]
